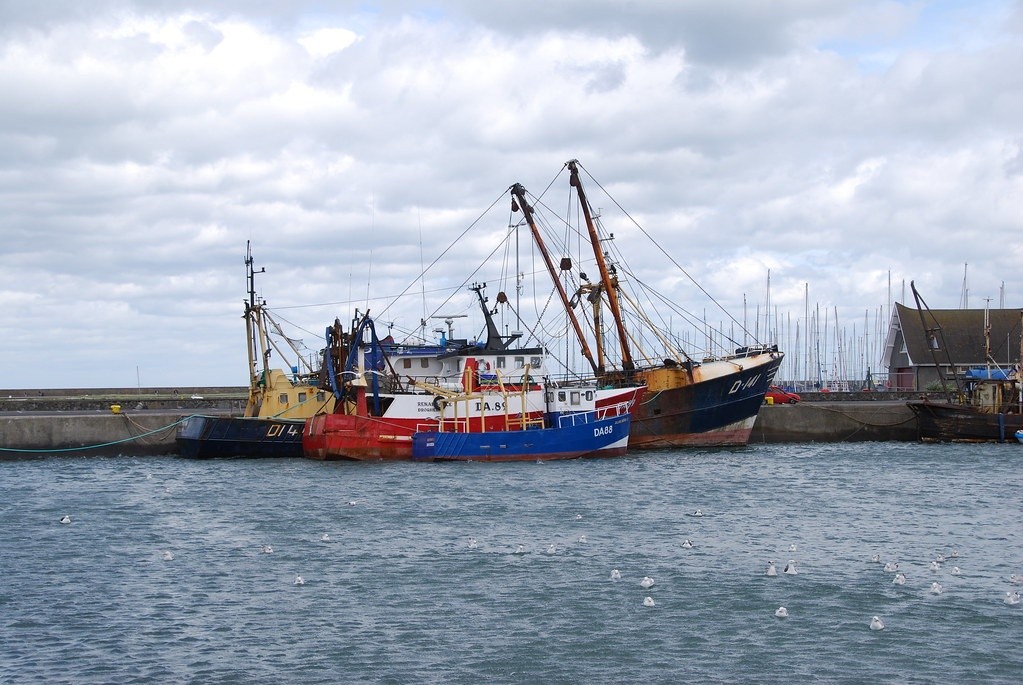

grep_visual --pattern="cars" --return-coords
[764,386,800,405]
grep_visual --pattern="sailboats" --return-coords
[172,159,893,463]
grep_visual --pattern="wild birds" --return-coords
[60,516,71,524]
[294,576,303,584]
[146,473,170,493]
[261,546,273,553]
[349,502,355,505]
[469,514,588,555]
[162,551,173,560]
[611,569,655,607]
[681,539,692,548]
[870,616,884,631]
[767,544,798,575]
[321,534,329,541]
[872,550,1021,605]
[694,509,702,516]
[775,606,788,618]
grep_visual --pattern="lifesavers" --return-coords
[174,390,178,394]
[475,359,491,375]
[434,396,447,410]
[155,390,160,394]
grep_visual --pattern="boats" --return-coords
[1015,430,1023,444]
[906,278,1023,444]
